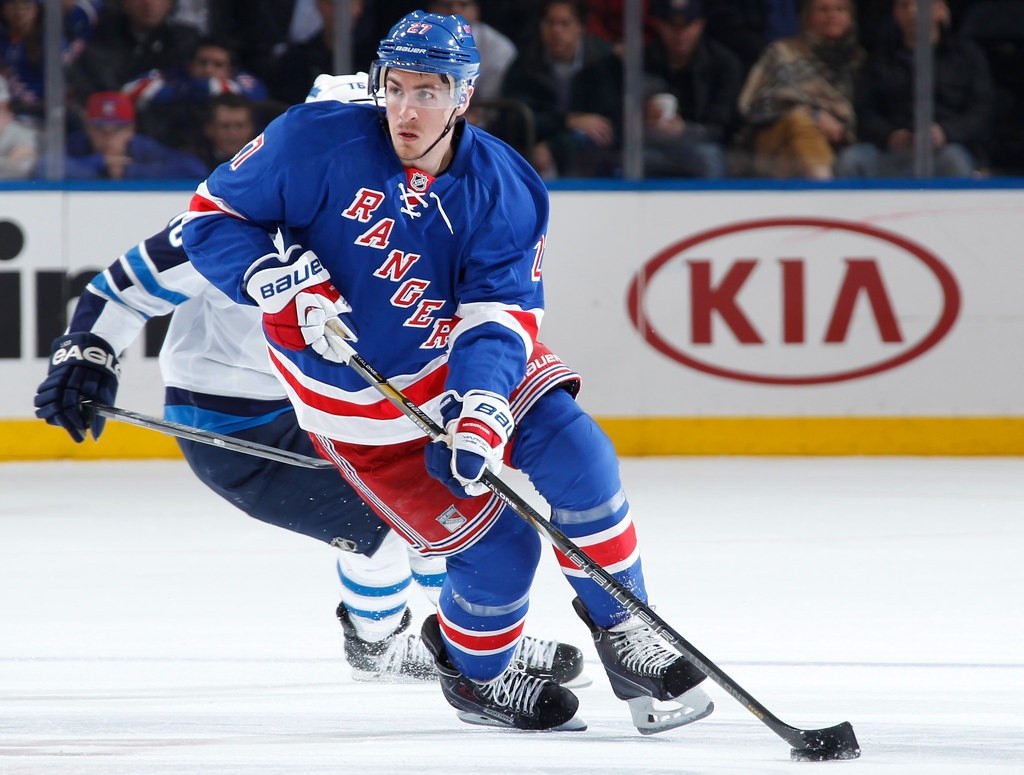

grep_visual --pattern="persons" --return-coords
[351,0,625,179]
[0,0,337,182]
[182,8,715,736]
[35,68,450,683]
[642,0,1001,180]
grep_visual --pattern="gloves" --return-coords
[34,332,118,443]
[244,250,361,366]
[424,394,515,498]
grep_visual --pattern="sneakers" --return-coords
[418,613,587,732]
[572,596,715,735]
[505,635,596,689]
[336,602,440,683]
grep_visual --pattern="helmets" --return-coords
[374,10,480,103]
[305,72,385,107]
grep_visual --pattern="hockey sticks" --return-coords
[324,323,861,755]
[75,393,337,470]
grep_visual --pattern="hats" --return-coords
[84,92,134,124]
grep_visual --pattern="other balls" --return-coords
[790,745,835,762]
